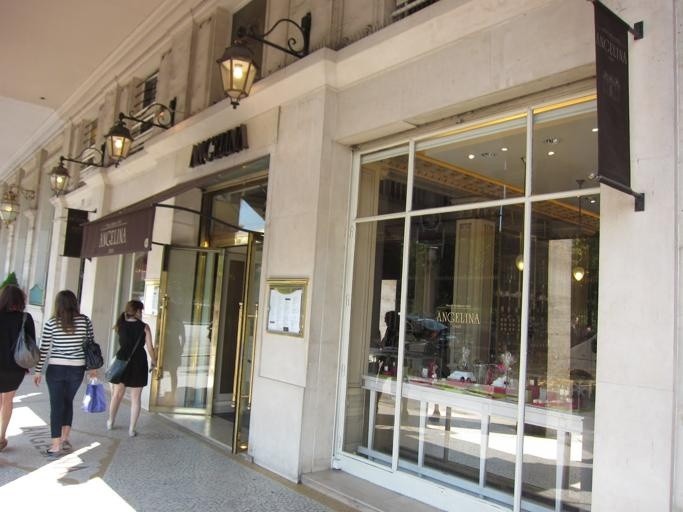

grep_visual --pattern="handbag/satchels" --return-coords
[104,354,129,383]
[81,375,107,413]
[80,315,104,371]
[13,312,41,369]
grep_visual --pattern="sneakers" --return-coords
[62,438,72,450]
[0,437,8,453]
[128,424,137,437]
[107,417,115,431]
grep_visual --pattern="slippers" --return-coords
[44,445,60,454]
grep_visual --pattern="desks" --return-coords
[361,371,594,512]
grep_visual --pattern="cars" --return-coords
[567,333,596,406]
[377,316,508,376]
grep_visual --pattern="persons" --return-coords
[0,284,35,451]
[33,290,97,453]
[374,312,409,425]
[157,301,186,393]
[106,300,157,437]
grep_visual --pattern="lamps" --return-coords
[0,183,35,229]
[47,141,106,199]
[103,96,178,169]
[514,158,524,270]
[217,10,312,110]
[571,178,585,282]
